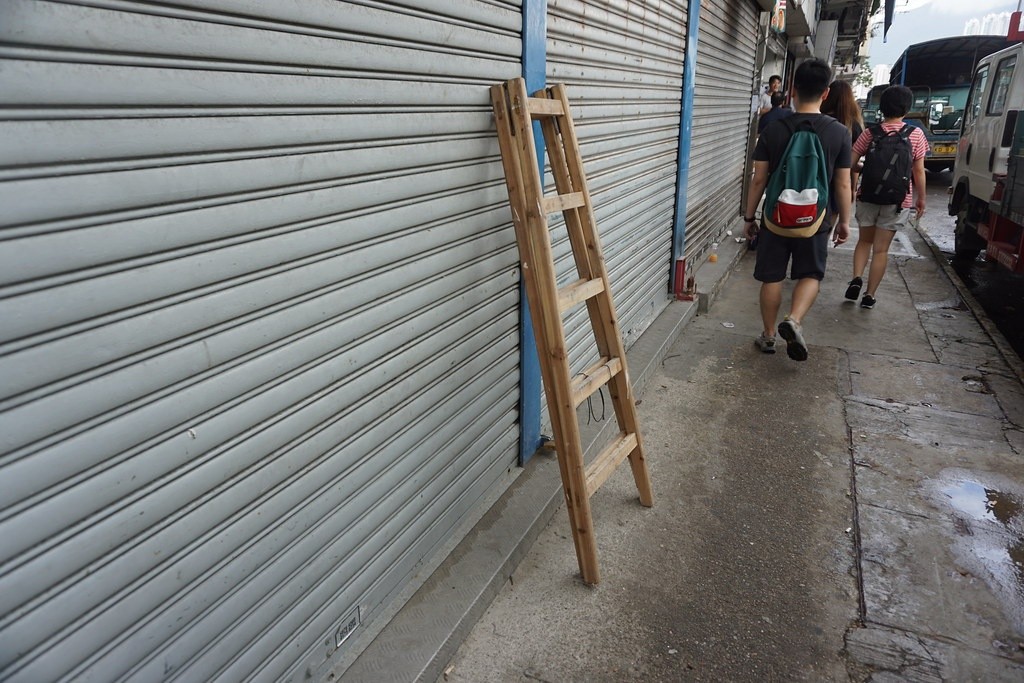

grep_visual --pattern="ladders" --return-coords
[490,78,655,588]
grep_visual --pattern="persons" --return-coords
[758,91,793,135]
[761,75,782,114]
[820,80,865,229]
[845,85,930,308]
[742,60,852,363]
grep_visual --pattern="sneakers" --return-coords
[754,331,777,353]
[844,277,863,301]
[778,315,808,361]
[861,295,877,309]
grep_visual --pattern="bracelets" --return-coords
[744,216,756,223]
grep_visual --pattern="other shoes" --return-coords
[748,233,758,251]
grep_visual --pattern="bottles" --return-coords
[710,243,718,262]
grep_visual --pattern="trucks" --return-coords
[946,40,1024,280]
[888,35,1024,172]
[856,84,890,129]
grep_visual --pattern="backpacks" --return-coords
[858,123,917,206]
[763,114,838,240]
